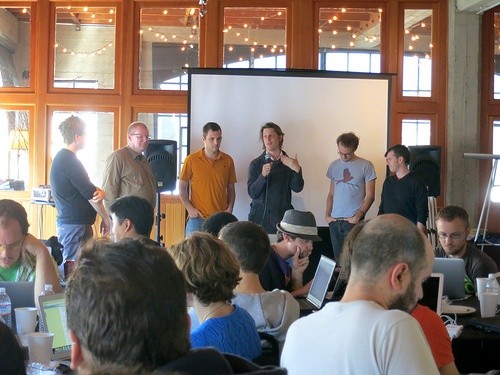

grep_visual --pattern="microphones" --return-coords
[265,154,271,175]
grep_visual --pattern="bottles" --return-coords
[0,287,13,328]
[485,273,498,294]
[38,283,57,331]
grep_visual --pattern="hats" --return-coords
[276,210,318,240]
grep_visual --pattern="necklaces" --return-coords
[202,302,226,323]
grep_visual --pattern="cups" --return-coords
[14,307,37,335]
[475,277,492,301]
[26,332,55,366]
[479,292,498,318]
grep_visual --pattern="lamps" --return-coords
[6,129,30,190]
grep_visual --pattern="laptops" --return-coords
[417,258,466,317]
[298,254,347,311]
[0,280,73,367]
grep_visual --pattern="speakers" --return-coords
[144,139,177,193]
[408,146,441,197]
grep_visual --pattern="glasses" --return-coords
[338,151,352,156]
[130,134,152,139]
[437,230,466,240]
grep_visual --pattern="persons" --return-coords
[101,121,157,242]
[280,214,441,375]
[167,231,263,361]
[110,195,153,244]
[50,115,111,281]
[64,232,288,375]
[0,199,61,315]
[339,219,458,375]
[178,123,237,238]
[201,211,239,237]
[247,122,305,234]
[325,132,376,267]
[434,205,498,289]
[218,221,301,350]
[263,209,323,300]
[377,144,430,238]
